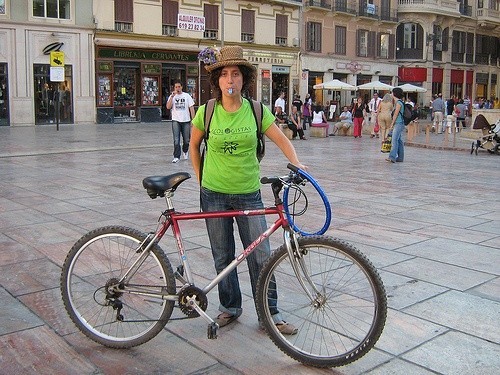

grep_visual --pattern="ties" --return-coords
[374,100,377,111]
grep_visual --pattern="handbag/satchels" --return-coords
[380,139,392,153]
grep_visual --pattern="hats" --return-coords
[204,46,256,73]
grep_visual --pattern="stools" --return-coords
[284,128,296,140]
[309,127,326,138]
[338,126,353,136]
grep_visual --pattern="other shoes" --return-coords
[183,151,190,160]
[171,157,181,163]
[385,158,404,163]
[212,311,238,328]
[258,319,299,336]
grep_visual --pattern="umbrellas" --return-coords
[357,81,395,98]
[390,83,427,98]
[313,79,359,100]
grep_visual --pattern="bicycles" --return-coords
[58,163,389,370]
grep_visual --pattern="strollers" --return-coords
[470,114,499,156]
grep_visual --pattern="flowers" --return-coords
[198,47,216,65]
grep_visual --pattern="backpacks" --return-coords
[397,100,417,126]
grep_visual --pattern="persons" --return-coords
[385,88,406,163]
[103,87,117,100]
[275,107,289,132]
[166,80,195,163]
[402,94,470,134]
[292,94,312,130]
[36,79,69,115]
[288,106,308,140]
[369,93,381,138]
[311,105,329,137]
[474,93,500,109]
[376,94,393,144]
[275,92,285,116]
[189,45,309,335]
[352,96,365,139]
[329,106,352,136]
